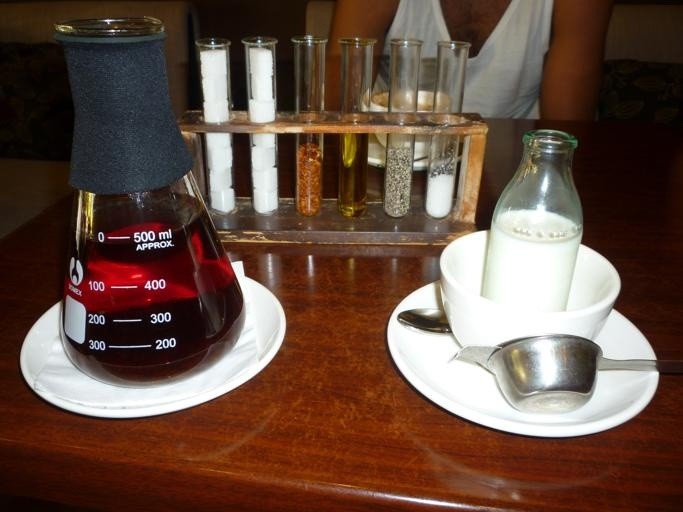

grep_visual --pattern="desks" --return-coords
[0,121,683,509]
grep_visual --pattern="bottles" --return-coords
[289,35,329,218]
[47,17,246,389]
[242,35,278,216]
[426,39,471,219]
[481,128,583,319]
[195,35,237,216]
[337,35,378,218]
[384,38,424,219]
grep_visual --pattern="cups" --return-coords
[371,90,450,160]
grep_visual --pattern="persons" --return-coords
[323,0,615,121]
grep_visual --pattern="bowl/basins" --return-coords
[437,228,626,358]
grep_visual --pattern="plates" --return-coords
[367,136,431,173]
[386,277,660,440]
[17,270,288,422]
